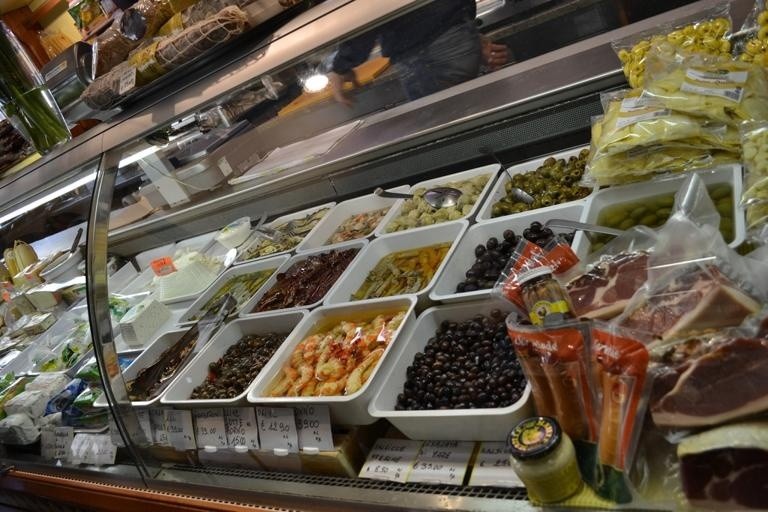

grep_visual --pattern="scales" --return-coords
[138,119,267,210]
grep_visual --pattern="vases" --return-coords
[0,21,71,156]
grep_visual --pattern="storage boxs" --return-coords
[93,146,746,442]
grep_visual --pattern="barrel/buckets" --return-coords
[38,246,84,283]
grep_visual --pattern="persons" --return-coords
[331,0,509,111]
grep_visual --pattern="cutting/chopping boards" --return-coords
[277,56,393,117]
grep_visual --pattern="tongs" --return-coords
[129,293,238,402]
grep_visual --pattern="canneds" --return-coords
[507,415,582,503]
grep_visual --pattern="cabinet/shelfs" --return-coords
[0,0,768,512]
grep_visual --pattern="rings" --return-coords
[487,57,492,63]
[491,51,495,56]
